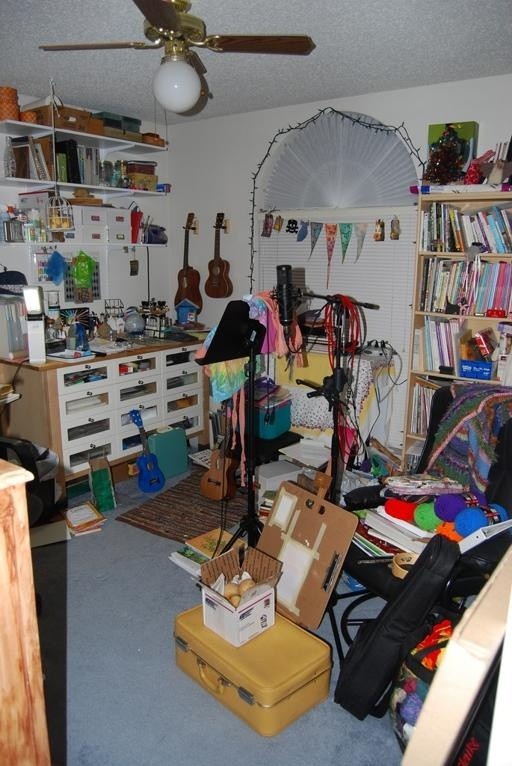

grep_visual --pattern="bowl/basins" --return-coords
[0,86,38,124]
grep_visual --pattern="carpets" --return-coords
[114,470,252,543]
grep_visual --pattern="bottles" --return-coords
[44,316,90,353]
[3,136,17,177]
[97,157,129,187]
[0,203,47,243]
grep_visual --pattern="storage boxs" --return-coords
[173,543,334,737]
[226,396,290,440]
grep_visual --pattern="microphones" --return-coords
[276,263,294,327]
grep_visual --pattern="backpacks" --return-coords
[379,474,464,504]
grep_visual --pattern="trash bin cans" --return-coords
[26,444,59,528]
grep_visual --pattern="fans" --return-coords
[35,0,318,76]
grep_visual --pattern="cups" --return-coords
[48,290,58,306]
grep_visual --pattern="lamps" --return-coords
[151,37,203,113]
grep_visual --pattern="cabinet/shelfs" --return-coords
[0,93,171,247]
[2,335,210,505]
[401,183,512,478]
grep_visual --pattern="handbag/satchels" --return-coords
[389,614,458,754]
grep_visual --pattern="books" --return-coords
[58,501,108,537]
[166,524,250,579]
[30,520,72,550]
[252,438,332,525]
[10,131,102,185]
[188,449,213,470]
[406,132,512,474]
[352,507,431,560]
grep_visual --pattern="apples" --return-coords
[224,579,256,608]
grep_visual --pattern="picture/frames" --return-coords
[62,500,108,531]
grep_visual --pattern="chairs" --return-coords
[344,381,512,670]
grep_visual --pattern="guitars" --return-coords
[130,411,165,492]
[200,425,238,502]
[205,214,233,299]
[175,214,202,315]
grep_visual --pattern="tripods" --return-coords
[195,328,266,590]
[296,287,380,670]
